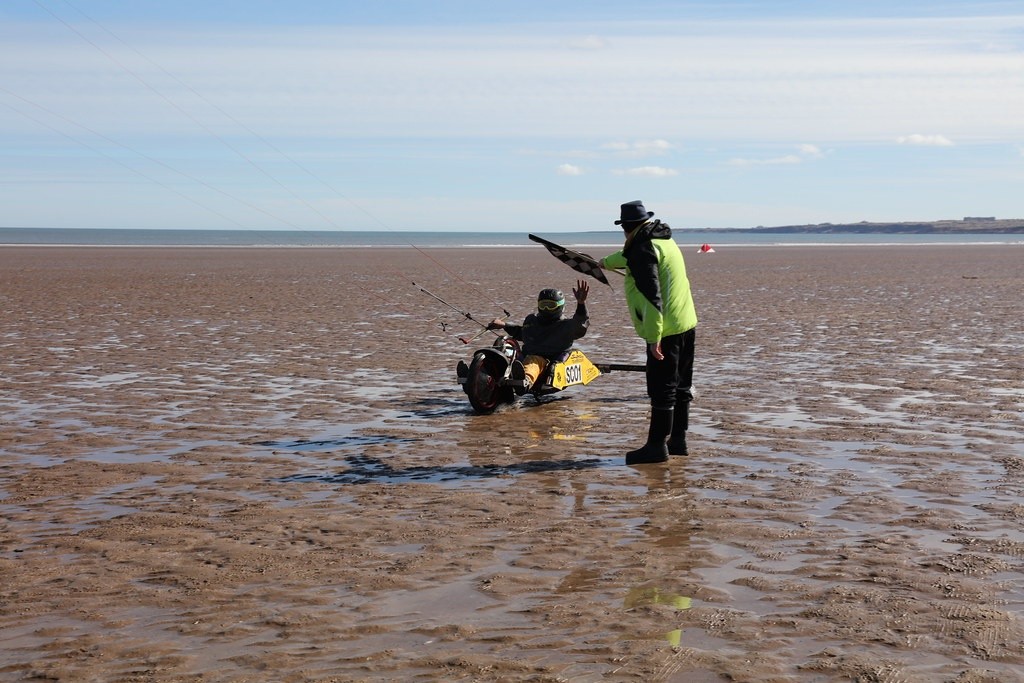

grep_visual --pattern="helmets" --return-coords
[537,288,565,319]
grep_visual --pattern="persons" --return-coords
[597,201,697,464]
[490,280,589,397]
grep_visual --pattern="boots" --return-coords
[666,400,690,456]
[626,406,675,465]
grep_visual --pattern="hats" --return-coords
[614,199,654,226]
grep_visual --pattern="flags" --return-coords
[542,243,610,287]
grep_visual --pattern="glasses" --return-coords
[538,299,565,310]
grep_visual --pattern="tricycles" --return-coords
[458,335,581,411]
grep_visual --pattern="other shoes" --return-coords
[511,360,526,397]
[456,359,470,395]
